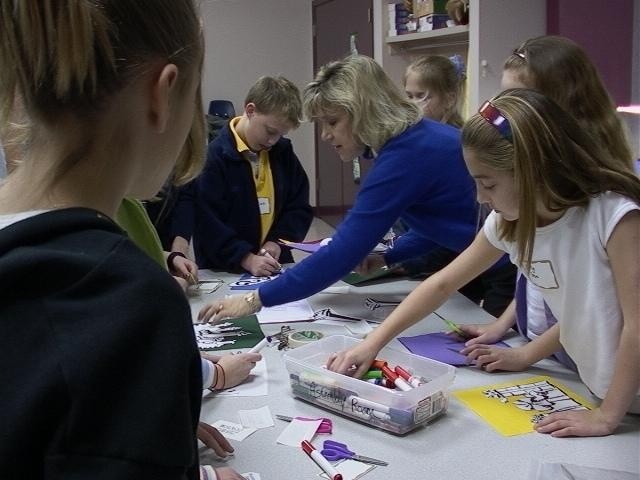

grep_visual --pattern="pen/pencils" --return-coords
[260,248,285,274]
[302,440,342,480]
[248,335,272,354]
[290,360,425,424]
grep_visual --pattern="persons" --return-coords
[447,34,640,371]
[330,90,640,437]
[197,53,518,334]
[391,54,467,272]
[193,74,315,277]
[114,169,263,392]
[0,0,211,480]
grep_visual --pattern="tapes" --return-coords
[287,329,326,348]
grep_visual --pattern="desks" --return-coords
[180,237,639,480]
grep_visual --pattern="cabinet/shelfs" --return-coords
[373,0,547,122]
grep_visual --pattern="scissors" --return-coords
[321,440,388,466]
[275,414,332,433]
[433,311,466,336]
